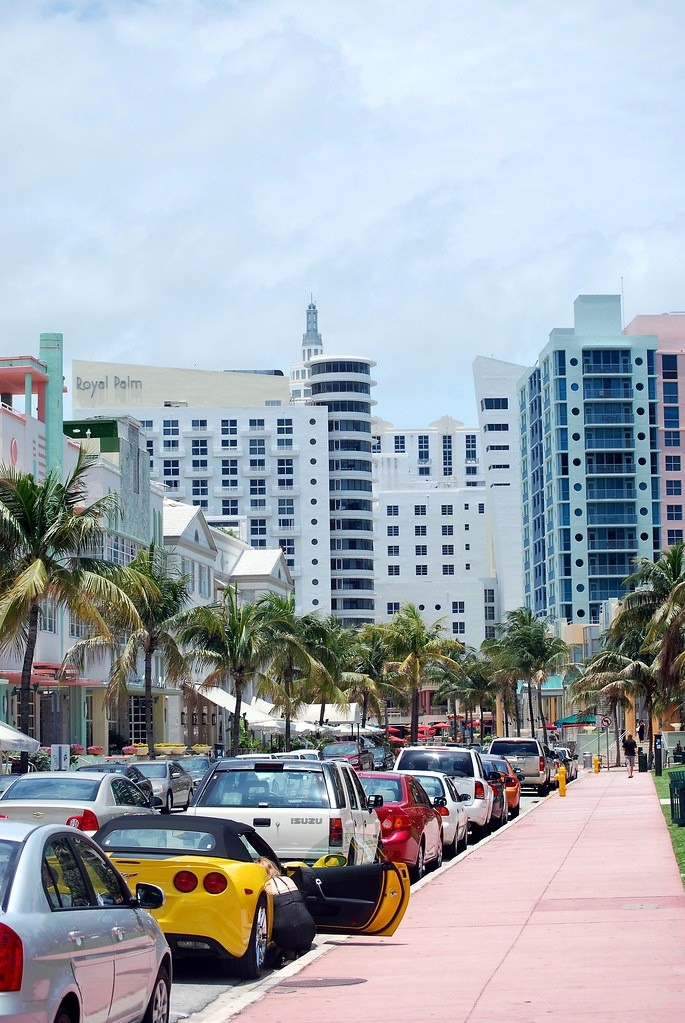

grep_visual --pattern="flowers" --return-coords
[124,746,135,748]
[70,744,84,753]
[87,745,104,751]
[194,743,208,747]
[153,742,186,747]
[133,743,148,747]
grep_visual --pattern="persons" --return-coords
[236,771,262,801]
[622,735,637,778]
[636,718,647,743]
[252,856,316,971]
[548,729,559,742]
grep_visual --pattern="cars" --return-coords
[480,753,526,819]
[0,774,25,797]
[0,816,174,1023]
[362,747,398,771]
[160,756,213,790]
[126,760,195,817]
[481,760,509,833]
[353,770,448,886]
[235,749,324,762]
[383,771,473,861]
[539,742,579,791]
[74,763,156,806]
[0,771,164,839]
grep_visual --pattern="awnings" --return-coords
[188,681,276,724]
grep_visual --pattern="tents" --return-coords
[255,695,362,747]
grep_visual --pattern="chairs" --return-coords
[242,780,270,804]
[424,787,435,796]
[197,834,212,849]
[413,760,428,770]
[43,866,74,908]
[375,790,395,801]
[109,838,139,846]
[453,761,465,771]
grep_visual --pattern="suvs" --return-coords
[481,737,550,797]
[391,746,502,844]
[185,758,384,869]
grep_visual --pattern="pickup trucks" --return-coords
[320,741,375,773]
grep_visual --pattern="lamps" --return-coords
[181,711,186,725]
[192,713,196,725]
[212,713,217,725]
[202,713,206,725]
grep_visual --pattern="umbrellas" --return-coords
[0,720,41,755]
[248,718,386,747]
[538,711,600,760]
[377,719,490,749]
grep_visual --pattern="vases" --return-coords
[73,749,83,754]
[122,747,137,755]
[171,747,188,754]
[88,749,105,755]
[137,747,149,755]
[153,747,172,754]
[191,746,212,755]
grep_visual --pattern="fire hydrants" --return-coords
[553,764,567,797]
[592,756,602,775]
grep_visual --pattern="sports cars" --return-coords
[41,814,411,980]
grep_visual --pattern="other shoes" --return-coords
[294,950,311,959]
[272,952,285,969]
[629,775,633,778]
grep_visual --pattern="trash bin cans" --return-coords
[582,751,592,772]
[669,770,685,827]
[638,753,647,772]
